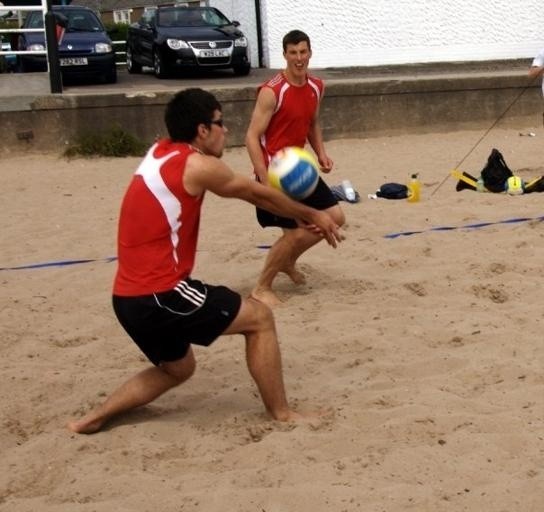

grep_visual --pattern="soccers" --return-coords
[268,146,319,198]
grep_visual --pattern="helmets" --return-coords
[504,176,524,195]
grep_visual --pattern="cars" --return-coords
[0,5,116,84]
[126,7,251,79]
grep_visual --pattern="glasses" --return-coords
[212,119,223,127]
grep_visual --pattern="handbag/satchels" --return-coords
[380,182,408,199]
[481,148,513,193]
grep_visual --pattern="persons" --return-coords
[69,90,346,435]
[528,46,544,119]
[249,29,345,308]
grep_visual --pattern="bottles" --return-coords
[408,174,421,204]
[341,179,356,203]
